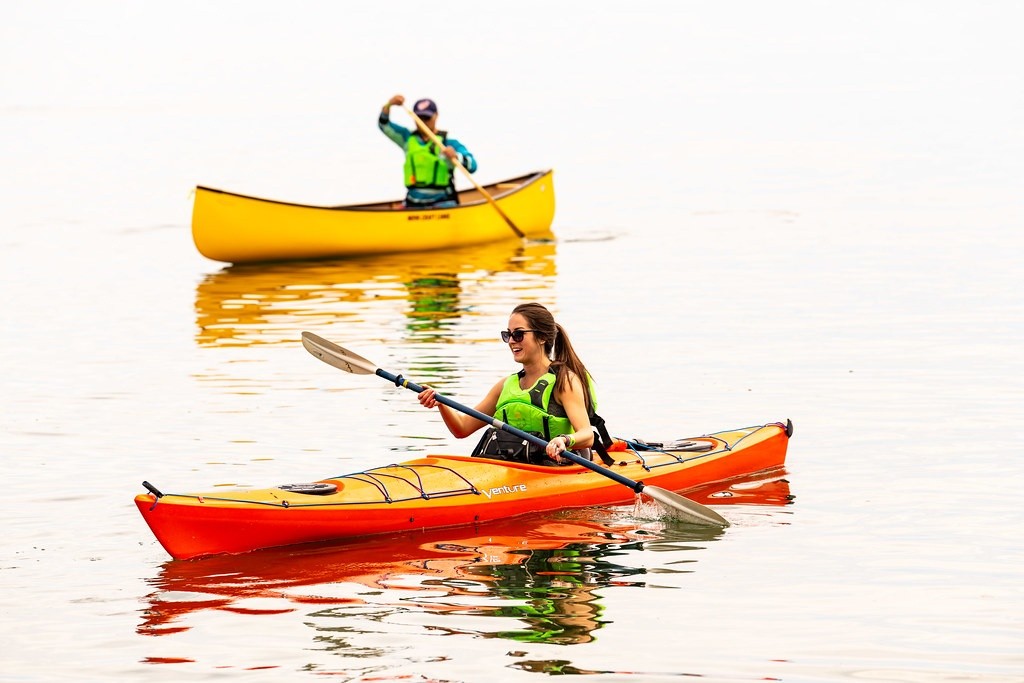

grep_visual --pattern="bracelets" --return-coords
[559,433,575,449]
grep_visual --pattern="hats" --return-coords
[413,99,437,117]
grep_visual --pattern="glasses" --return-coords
[501,329,539,343]
[416,116,433,122]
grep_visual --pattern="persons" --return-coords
[378,95,477,210]
[418,303,598,467]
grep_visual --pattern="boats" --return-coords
[132,416,795,563]
[189,168,557,264]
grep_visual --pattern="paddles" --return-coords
[403,104,526,238]
[301,329,731,529]
